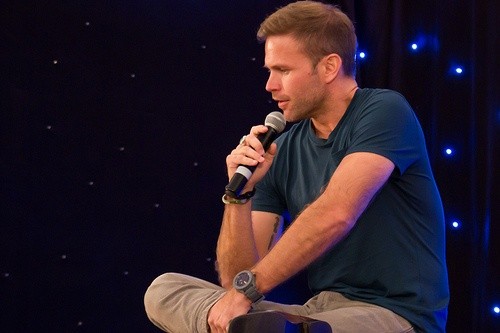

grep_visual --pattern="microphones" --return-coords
[225,112,286,198]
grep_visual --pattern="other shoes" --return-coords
[226,310,332,333]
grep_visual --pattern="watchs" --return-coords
[233,270,265,305]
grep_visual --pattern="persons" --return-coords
[143,0,450,333]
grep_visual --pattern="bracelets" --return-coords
[233,188,257,200]
[222,194,250,206]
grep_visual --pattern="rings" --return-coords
[240,136,248,146]
